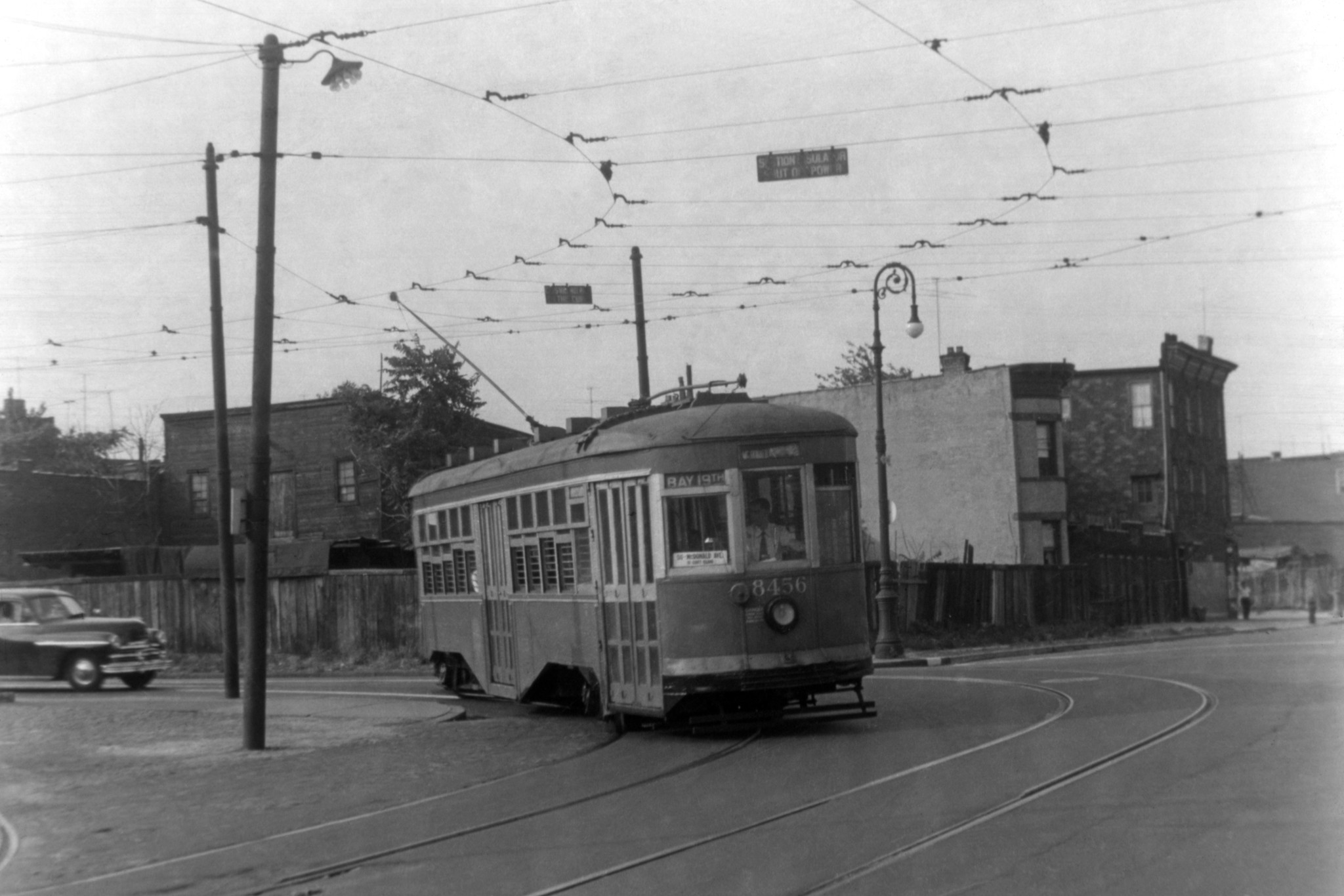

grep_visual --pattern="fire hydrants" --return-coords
[1308,598,1317,623]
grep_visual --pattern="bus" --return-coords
[389,292,877,735]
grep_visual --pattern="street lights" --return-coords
[874,262,924,659]
[243,33,363,748]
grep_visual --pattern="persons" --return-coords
[746,499,805,561]
[1239,581,1252,620]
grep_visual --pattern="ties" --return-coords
[760,532,767,561]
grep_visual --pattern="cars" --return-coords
[0,589,172,692]
